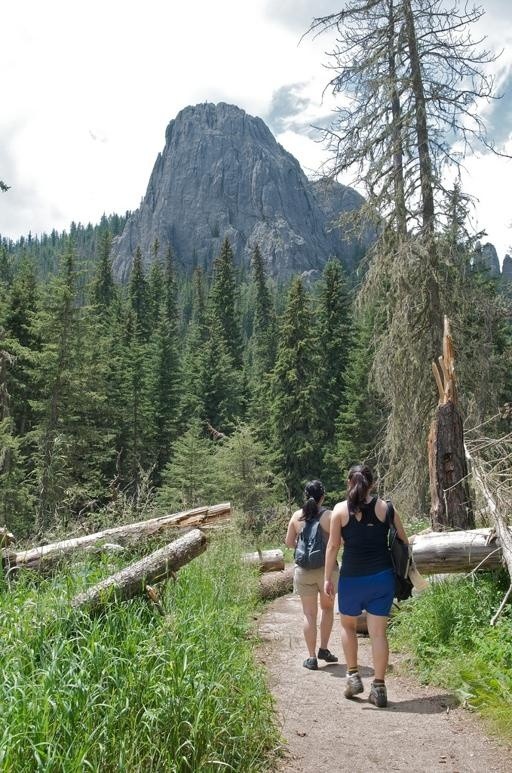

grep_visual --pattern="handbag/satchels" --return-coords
[389,528,428,601]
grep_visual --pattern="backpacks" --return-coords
[294,508,329,569]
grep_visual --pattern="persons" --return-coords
[322,465,417,709]
[283,480,339,670]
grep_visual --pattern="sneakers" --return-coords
[302,658,317,670]
[318,648,338,662]
[368,683,387,707]
[343,670,363,698]
